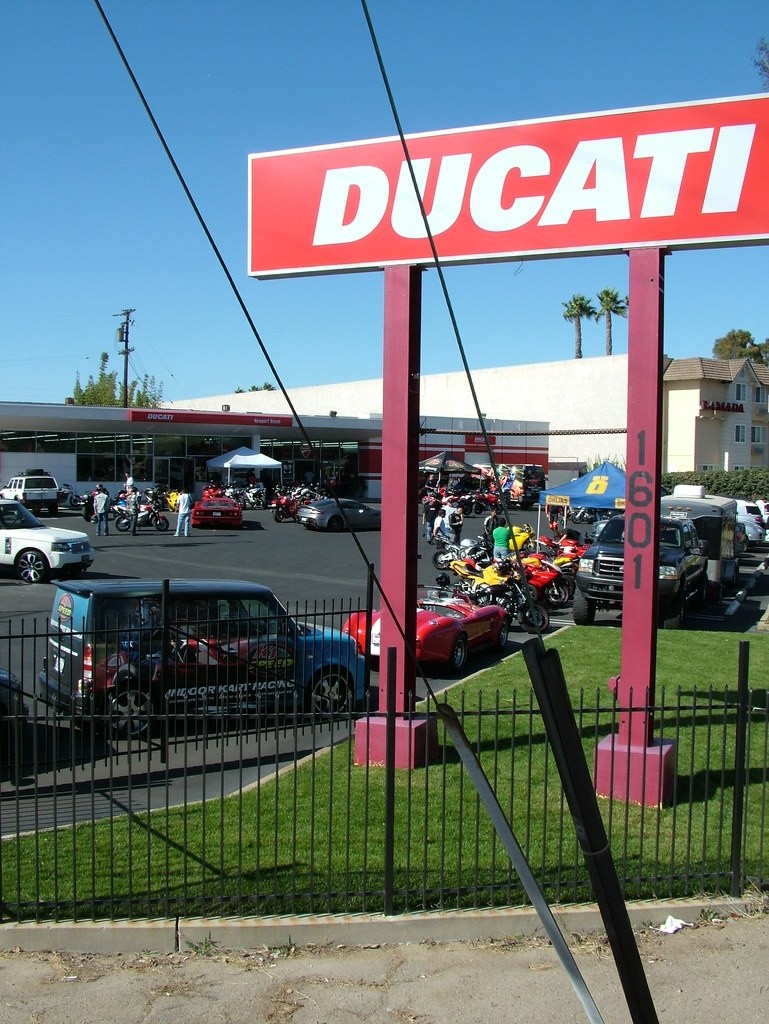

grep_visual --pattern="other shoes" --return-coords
[428,541,434,545]
[174,534,179,537]
[131,533,140,536]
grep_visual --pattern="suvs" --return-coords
[574,514,710,630]
[0,499,96,580]
[0,474,61,514]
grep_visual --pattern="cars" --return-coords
[43,578,366,721]
[299,498,381,532]
[735,500,769,552]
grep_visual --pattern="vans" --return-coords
[662,485,741,601]
[471,464,545,510]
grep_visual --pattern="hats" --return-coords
[430,497,436,502]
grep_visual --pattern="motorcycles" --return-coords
[429,485,604,633]
[79,484,110,522]
[113,483,194,533]
[204,483,326,523]
[58,483,80,506]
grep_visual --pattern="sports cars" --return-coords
[343,591,513,674]
[192,498,244,528]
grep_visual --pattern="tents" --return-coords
[536,461,671,554]
[205,446,282,487]
[419,450,482,488]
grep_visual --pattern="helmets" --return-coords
[96,484,103,490]
[131,487,138,492]
[435,573,451,586]
[495,562,507,575]
[91,517,98,524]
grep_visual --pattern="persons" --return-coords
[174,489,193,536]
[545,505,561,533]
[94,484,110,536]
[484,508,498,542]
[447,478,455,494]
[123,472,133,489]
[127,486,140,536]
[492,518,513,558]
[425,474,435,495]
[422,493,464,543]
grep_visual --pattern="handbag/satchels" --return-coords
[188,494,195,509]
